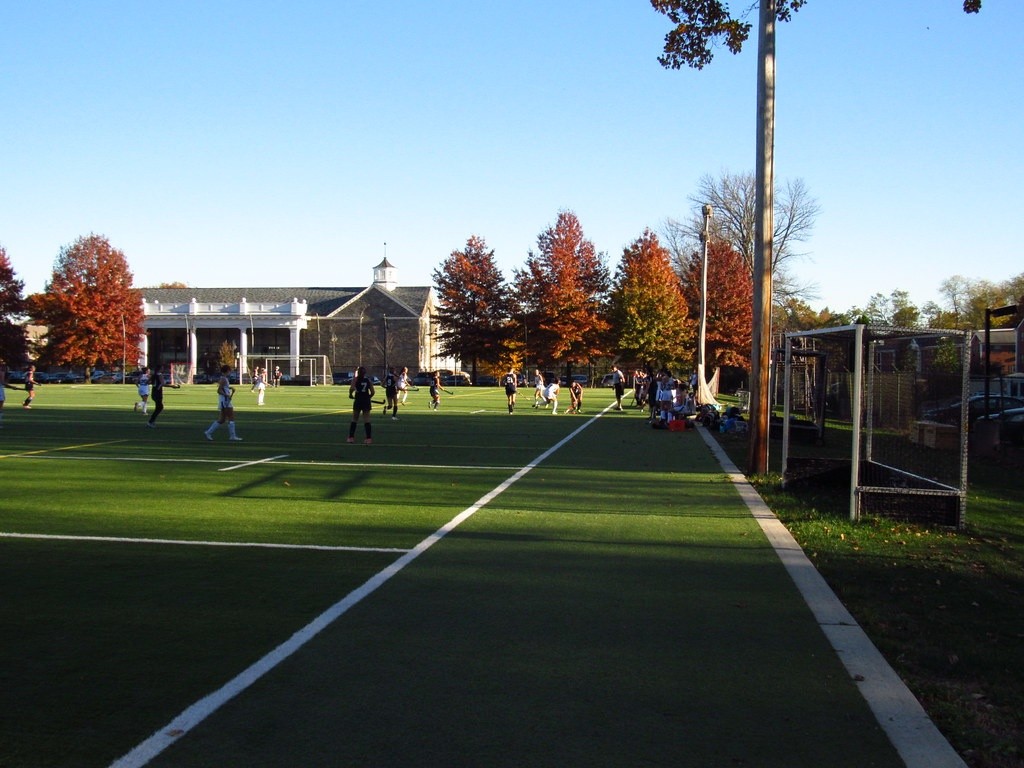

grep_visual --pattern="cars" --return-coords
[193,369,239,385]
[540,372,588,388]
[281,374,292,384]
[412,369,471,385]
[5,370,173,385]
[973,407,1024,442]
[921,396,1024,428]
[600,373,615,387]
[341,376,382,386]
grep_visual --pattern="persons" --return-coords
[611,365,625,410]
[134,365,177,428]
[503,367,517,414]
[346,366,375,443]
[23,365,37,409]
[429,371,444,411]
[381,367,410,421]
[273,366,282,387]
[534,370,560,414]
[0,358,16,415]
[205,365,243,440]
[569,380,583,414]
[632,367,748,440]
[251,366,270,405]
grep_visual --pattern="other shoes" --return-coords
[551,412,558,415]
[146,422,157,428]
[25,406,31,409]
[142,413,148,415]
[365,439,372,444]
[229,437,243,440]
[428,402,431,409]
[205,431,214,441]
[383,406,387,414]
[392,417,399,421]
[347,438,353,442]
[134,403,138,412]
[401,401,405,406]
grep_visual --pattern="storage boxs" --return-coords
[669,420,685,431]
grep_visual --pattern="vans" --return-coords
[332,373,355,384]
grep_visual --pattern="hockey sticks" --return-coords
[14,388,33,390]
[393,373,416,388]
[353,397,385,404]
[440,387,453,395]
[630,396,635,407]
[385,387,419,391]
[217,392,234,424]
[564,405,574,414]
[168,385,180,388]
[517,391,531,400]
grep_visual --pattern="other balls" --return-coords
[572,412,575,414]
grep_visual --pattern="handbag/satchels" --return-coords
[651,407,747,434]
